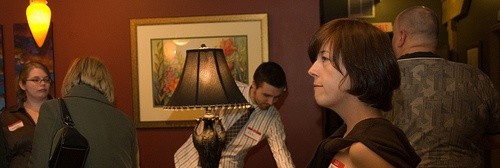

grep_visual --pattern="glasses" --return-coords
[25,78,52,84]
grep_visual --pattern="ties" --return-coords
[223,106,256,149]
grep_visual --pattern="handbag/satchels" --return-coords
[48,97,90,168]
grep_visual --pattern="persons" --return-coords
[379,6,500,168]
[0,62,53,168]
[26,57,140,168]
[173,61,296,168]
[304,18,421,168]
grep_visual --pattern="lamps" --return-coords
[25,0,51,48]
[162,43,253,168]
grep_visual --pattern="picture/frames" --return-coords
[464,41,482,69]
[129,13,270,128]
